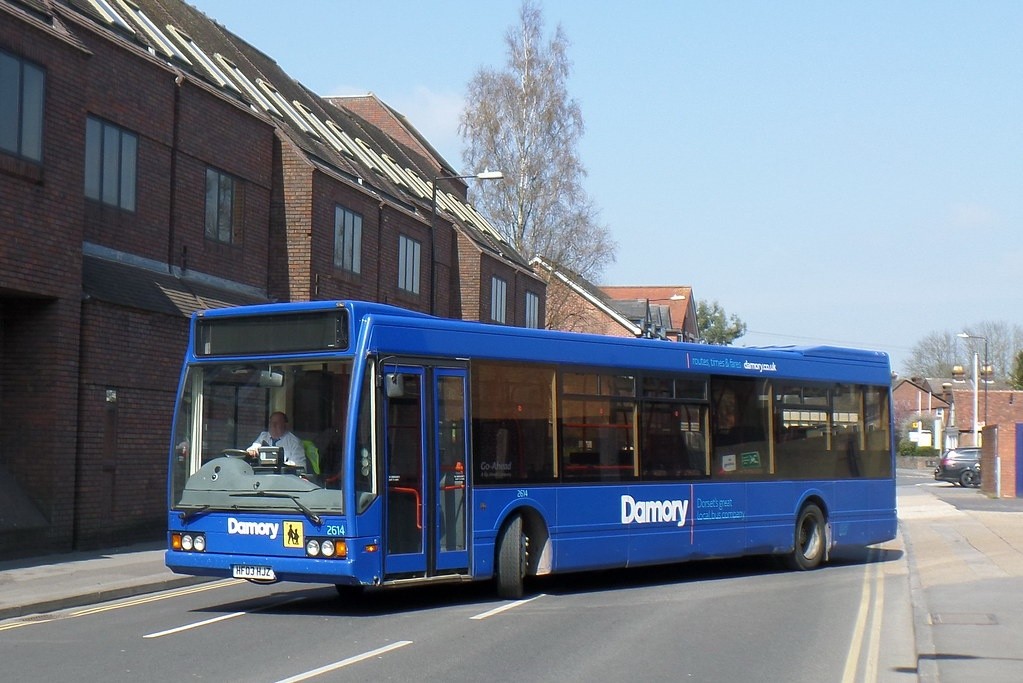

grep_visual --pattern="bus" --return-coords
[165,300,899,600]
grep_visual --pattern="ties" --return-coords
[269,438,280,446]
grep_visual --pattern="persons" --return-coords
[246,412,307,474]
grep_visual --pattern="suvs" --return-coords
[934,447,981,488]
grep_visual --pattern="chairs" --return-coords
[779,424,874,440]
[301,437,321,486]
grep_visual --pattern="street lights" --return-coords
[429,171,505,316]
[646,295,685,338]
[958,333,988,425]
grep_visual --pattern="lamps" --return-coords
[105,385,121,403]
